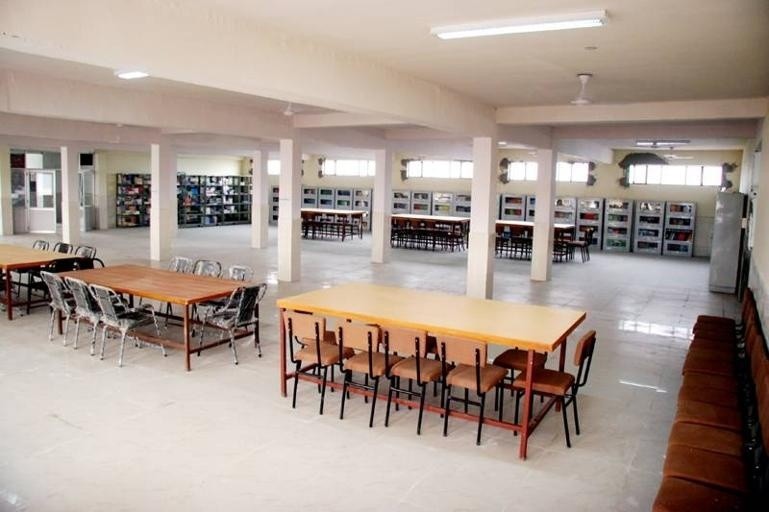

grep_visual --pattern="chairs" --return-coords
[283,309,456,435]
[652,287,769,512]
[437,333,508,445]
[0,240,268,367]
[391,216,469,252]
[301,211,367,242]
[495,223,594,262]
[494,346,548,403]
[512,330,594,446]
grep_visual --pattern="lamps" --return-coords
[429,10,607,40]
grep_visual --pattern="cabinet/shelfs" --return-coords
[392,191,471,217]
[177,175,252,228]
[269,184,372,231]
[501,194,577,223]
[709,191,744,295]
[576,198,695,257]
[116,173,151,228]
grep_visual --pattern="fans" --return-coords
[570,75,595,105]
[283,102,318,117]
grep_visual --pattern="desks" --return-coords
[276,284,587,461]
